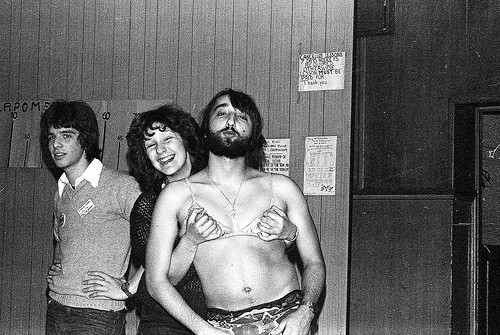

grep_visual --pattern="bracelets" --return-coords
[181,239,197,252]
[291,232,297,241]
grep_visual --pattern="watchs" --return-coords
[301,300,318,313]
[122,282,133,297]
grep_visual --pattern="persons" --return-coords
[39,100,145,335]
[125,104,297,335]
[145,89,325,335]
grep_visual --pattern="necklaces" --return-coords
[206,170,247,217]
